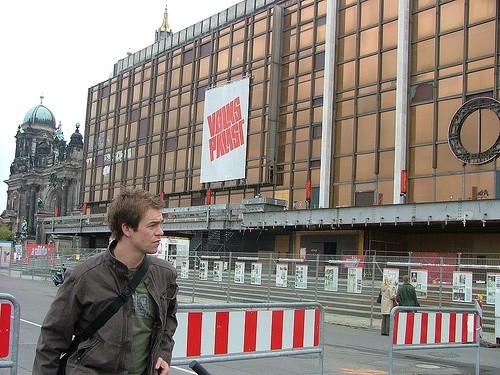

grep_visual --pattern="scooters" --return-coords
[51,263,67,286]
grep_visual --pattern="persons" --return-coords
[33,189,179,375]
[22,218,28,233]
[396,275,421,312]
[380,277,397,335]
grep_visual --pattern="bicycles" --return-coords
[189,359,211,375]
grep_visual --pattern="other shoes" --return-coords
[381,333,389,336]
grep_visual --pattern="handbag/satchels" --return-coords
[57,352,67,375]
[393,298,399,307]
[377,288,381,303]
